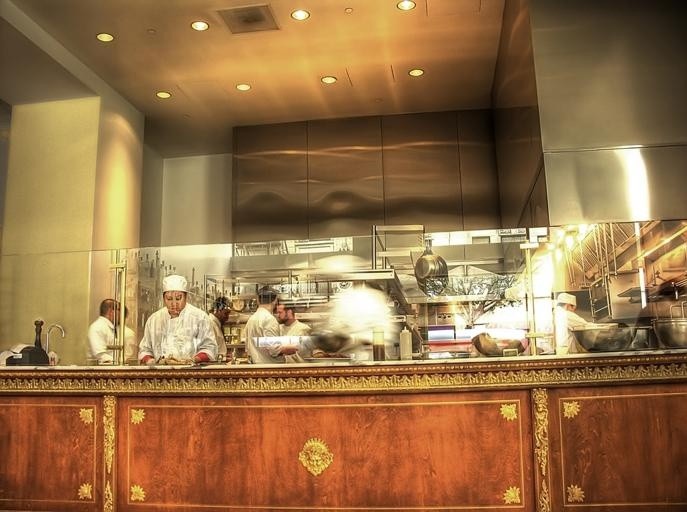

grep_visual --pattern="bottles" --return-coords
[400,326,413,360]
[373,326,385,361]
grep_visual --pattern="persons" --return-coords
[205,295,236,363]
[275,302,314,364]
[298,326,372,361]
[243,283,300,363]
[550,291,597,355]
[84,297,122,367]
[135,275,220,366]
[113,301,138,367]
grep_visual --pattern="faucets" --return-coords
[45,323,66,353]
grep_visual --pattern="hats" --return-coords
[559,293,576,305]
[162,275,188,293]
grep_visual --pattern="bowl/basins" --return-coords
[571,317,687,351]
[472,329,530,357]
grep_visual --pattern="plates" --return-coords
[304,353,356,362]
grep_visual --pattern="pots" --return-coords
[415,234,450,284]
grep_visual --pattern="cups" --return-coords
[224,334,239,345]
